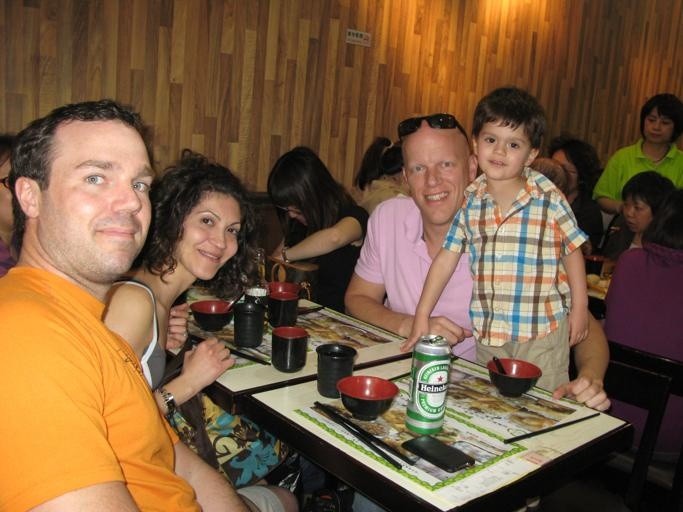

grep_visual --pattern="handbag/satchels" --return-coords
[266,255,320,303]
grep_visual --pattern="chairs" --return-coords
[247,191,321,300]
[569,338,682,511]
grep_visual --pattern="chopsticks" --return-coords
[185,331,272,368]
[503,412,601,447]
[313,397,415,475]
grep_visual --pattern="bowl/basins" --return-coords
[188,299,236,333]
[485,357,545,398]
[334,376,399,423]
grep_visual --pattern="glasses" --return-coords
[398,113,470,148]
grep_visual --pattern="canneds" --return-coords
[403,335,453,435]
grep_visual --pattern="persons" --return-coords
[363,95,683,462]
[267,146,369,315]
[400,86,589,390]
[0,98,339,511]
[344,120,609,411]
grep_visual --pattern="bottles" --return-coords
[254,247,266,286]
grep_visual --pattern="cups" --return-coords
[267,293,299,328]
[582,253,604,276]
[314,342,358,400]
[271,325,308,373]
[244,287,269,304]
[266,281,302,299]
[234,302,265,350]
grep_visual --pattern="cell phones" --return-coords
[401,434,475,472]
